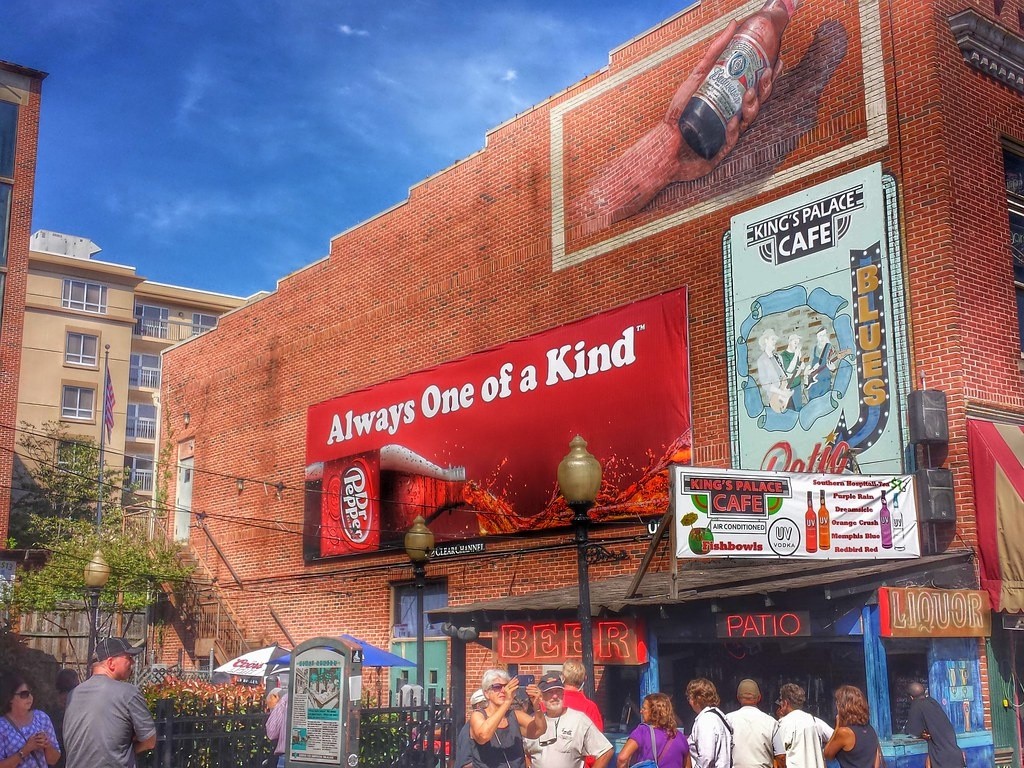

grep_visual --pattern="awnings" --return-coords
[967,418,1024,613]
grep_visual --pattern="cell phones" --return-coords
[516,675,535,686]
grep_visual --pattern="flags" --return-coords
[105,368,116,444]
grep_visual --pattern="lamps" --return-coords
[236,478,244,490]
[183,412,190,425]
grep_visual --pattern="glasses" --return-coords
[539,721,557,746]
[11,691,36,699]
[487,683,508,693]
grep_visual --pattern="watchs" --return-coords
[18,749,25,761]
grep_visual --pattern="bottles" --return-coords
[678,1,799,160]
[892,493,905,551]
[880,490,892,548]
[818,490,830,550]
[302,444,465,560]
[805,491,817,553]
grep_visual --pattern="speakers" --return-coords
[916,469,956,523]
[906,390,949,446]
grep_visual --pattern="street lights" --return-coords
[84,548,109,682]
[558,433,602,703]
[405,514,435,748]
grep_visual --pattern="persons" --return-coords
[617,692,692,768]
[455,658,615,768]
[686,678,735,768]
[823,685,881,768]
[775,682,835,768]
[62,637,157,768]
[424,696,449,740]
[265,692,288,768]
[38,668,81,768]
[725,679,787,768]
[0,668,61,768]
[905,682,967,768]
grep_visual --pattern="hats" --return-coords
[537,673,565,693]
[92,637,144,661]
[470,691,489,704]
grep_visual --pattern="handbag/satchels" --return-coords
[628,722,657,768]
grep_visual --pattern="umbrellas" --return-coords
[210,641,291,685]
[264,634,417,667]
